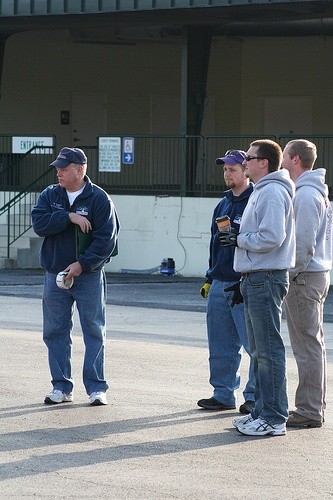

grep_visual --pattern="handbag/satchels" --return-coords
[75,224,119,260]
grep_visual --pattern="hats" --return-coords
[49,147,87,168]
[215,150,247,166]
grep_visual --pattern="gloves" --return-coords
[216,227,240,247]
[199,280,212,299]
[224,281,245,308]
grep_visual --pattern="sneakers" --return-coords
[89,391,108,405]
[238,415,286,436]
[44,389,73,403]
[232,413,255,427]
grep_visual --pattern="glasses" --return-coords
[224,150,245,160]
[244,154,271,165]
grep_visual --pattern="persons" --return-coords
[220,139,296,435]
[31,147,119,404]
[282,139,333,428]
[197,149,257,414]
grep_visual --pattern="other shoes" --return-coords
[289,410,324,422]
[239,400,255,414]
[197,397,236,410]
[286,413,322,427]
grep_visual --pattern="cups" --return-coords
[215,215,231,233]
